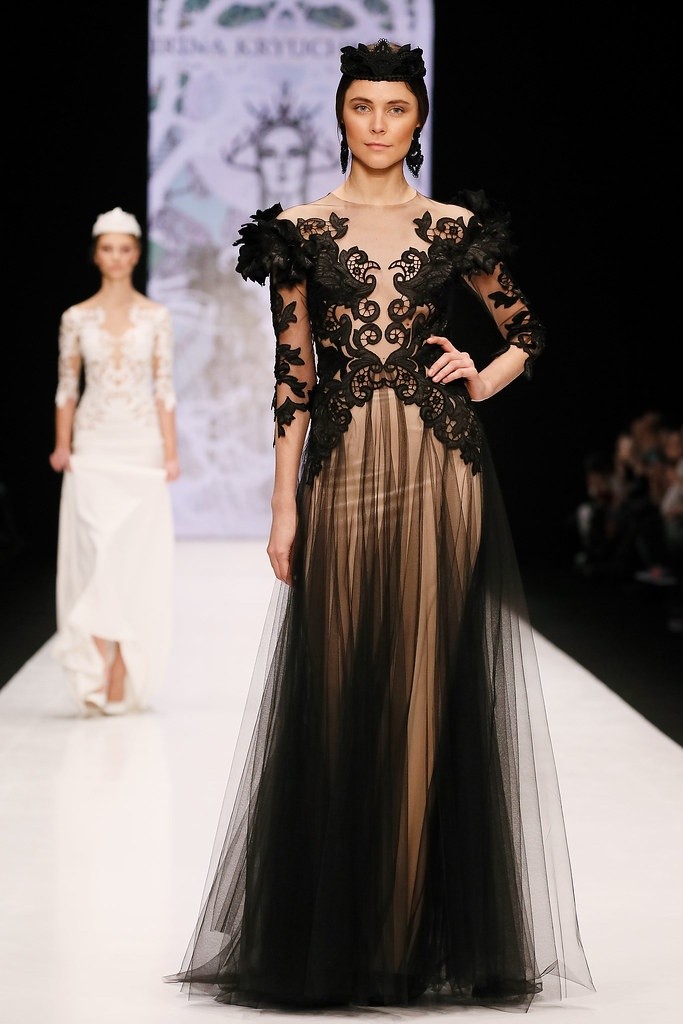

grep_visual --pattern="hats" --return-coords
[93,207,141,239]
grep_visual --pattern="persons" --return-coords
[161,38,593,1017]
[51,206,183,715]
[576,413,683,632]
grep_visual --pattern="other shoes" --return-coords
[83,686,128,714]
